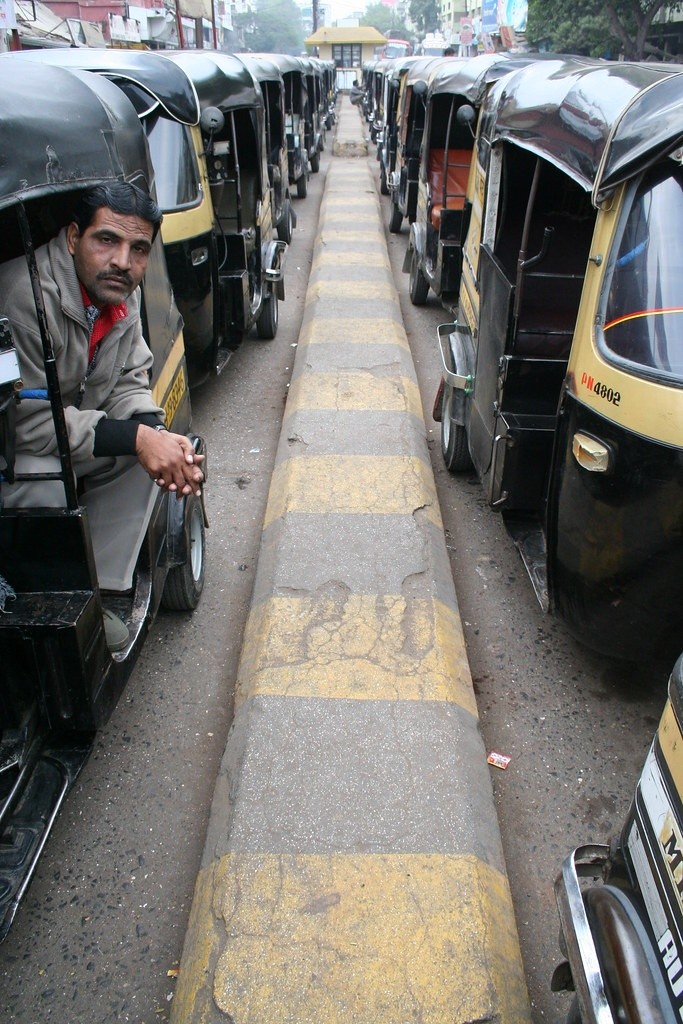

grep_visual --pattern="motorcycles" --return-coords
[0,56,210,949]
[2,48,340,386]
[548,647,683,1024]
[352,50,599,320]
[429,53,682,649]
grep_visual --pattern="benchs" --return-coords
[428,147,472,231]
[217,168,259,255]
[408,128,425,180]
[498,211,598,358]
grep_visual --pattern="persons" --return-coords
[0,181,205,653]
[444,48,454,56]
